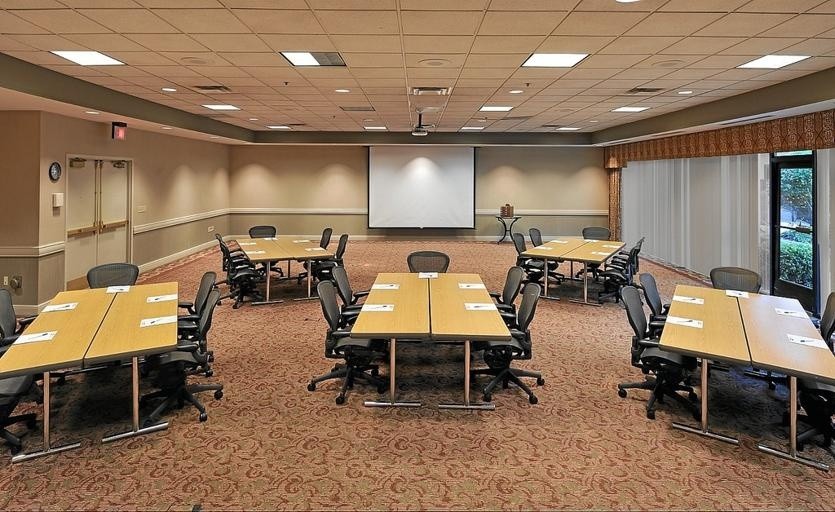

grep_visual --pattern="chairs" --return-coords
[619,266,701,421]
[710,268,762,293]
[85,264,139,288]
[307,264,388,405]
[408,251,451,274]
[511,223,648,311]
[781,291,834,451]
[213,215,348,318]
[471,267,545,405]
[0,263,68,453]
[142,271,224,423]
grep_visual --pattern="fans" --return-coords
[368,147,476,230]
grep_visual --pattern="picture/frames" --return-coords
[492,214,522,246]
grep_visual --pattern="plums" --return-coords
[411,128,428,136]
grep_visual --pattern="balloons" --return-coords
[49,162,62,180]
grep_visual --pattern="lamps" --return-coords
[213,215,348,318]
[511,223,648,311]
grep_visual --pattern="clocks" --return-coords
[49,162,62,180]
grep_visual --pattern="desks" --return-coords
[492,214,522,246]
[351,273,513,411]
[658,285,835,471]
[4,280,178,463]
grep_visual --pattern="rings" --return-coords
[474,305,490,307]
[683,298,696,301]
[801,339,816,342]
[58,306,70,309]
[156,297,164,301]
[111,287,124,290]
[784,312,795,314]
[675,320,692,323]
[149,318,161,324]
